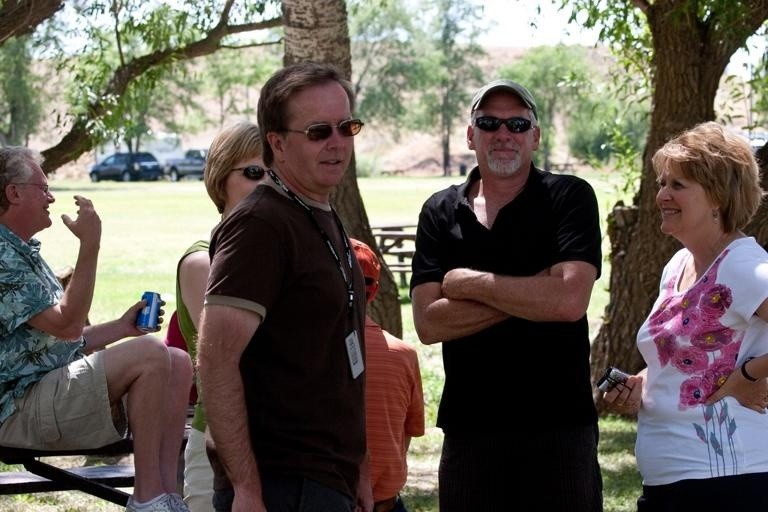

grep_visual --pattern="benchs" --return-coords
[1,404,203,512]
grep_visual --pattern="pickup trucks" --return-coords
[164,147,209,185]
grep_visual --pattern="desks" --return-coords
[370,220,420,289]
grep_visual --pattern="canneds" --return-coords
[136,291,161,333]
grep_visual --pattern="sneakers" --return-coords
[126,492,190,512]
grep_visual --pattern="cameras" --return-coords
[597,366,629,392]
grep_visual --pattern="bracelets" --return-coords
[741,356,761,383]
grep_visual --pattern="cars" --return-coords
[87,151,162,183]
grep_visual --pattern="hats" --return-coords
[471,79,536,115]
[348,237,381,292]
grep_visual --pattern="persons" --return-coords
[194,64,365,512]
[0,144,200,512]
[407,79,600,512]
[346,236,423,512]
[174,118,288,512]
[603,116,768,509]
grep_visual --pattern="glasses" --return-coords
[288,119,364,141]
[474,116,531,134]
[231,165,268,180]
